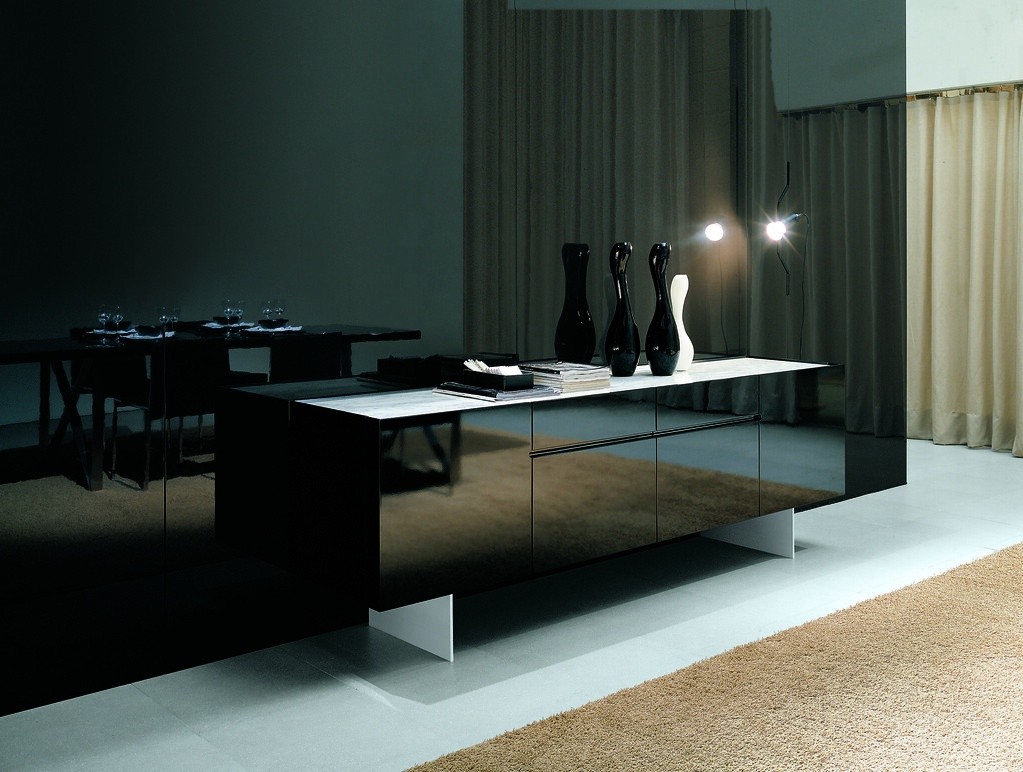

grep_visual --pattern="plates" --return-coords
[245,325,304,333]
[200,321,254,329]
[119,331,177,340]
[86,328,137,335]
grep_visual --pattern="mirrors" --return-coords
[0,0,909,718]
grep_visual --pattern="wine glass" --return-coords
[95,304,124,349]
[261,297,286,326]
[157,303,178,343]
[224,296,246,338]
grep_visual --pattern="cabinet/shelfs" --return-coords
[210,354,555,622]
[289,355,848,660]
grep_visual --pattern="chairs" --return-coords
[109,334,246,490]
[268,327,347,385]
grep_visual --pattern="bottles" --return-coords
[605,242,641,377]
[555,241,596,364]
[669,275,695,371]
[646,243,676,375]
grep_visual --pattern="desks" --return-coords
[67,322,424,491]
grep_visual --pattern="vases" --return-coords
[554,243,597,364]
[604,242,641,377]
[644,241,679,376]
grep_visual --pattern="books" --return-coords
[518,363,611,392]
[433,382,560,401]
